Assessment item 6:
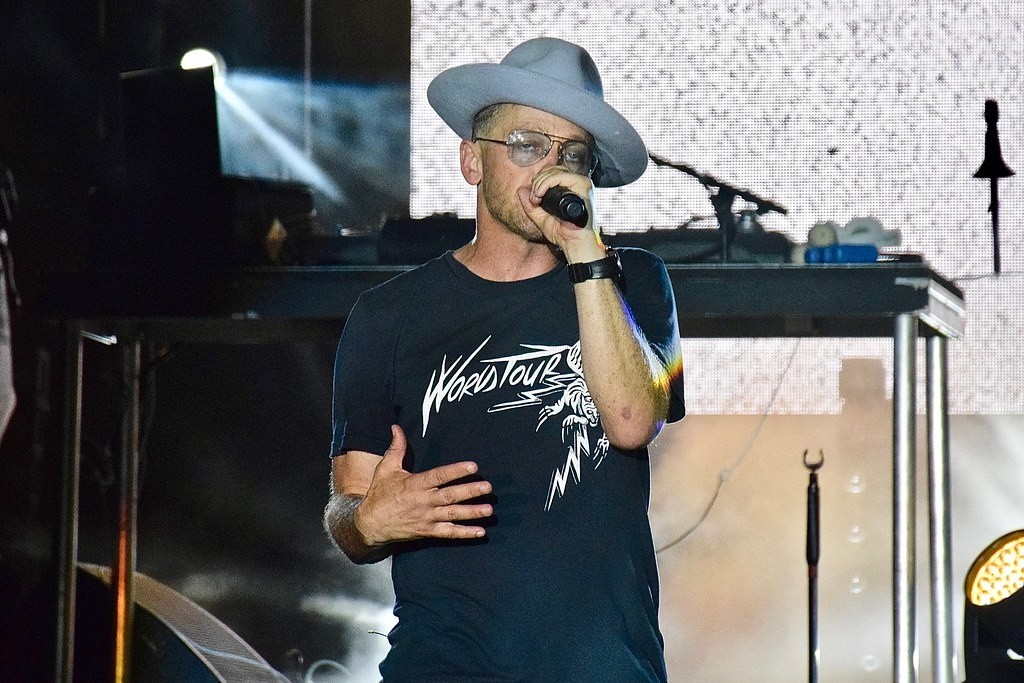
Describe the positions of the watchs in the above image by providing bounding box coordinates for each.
[568,245,623,284]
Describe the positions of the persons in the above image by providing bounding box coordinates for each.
[324,38,685,683]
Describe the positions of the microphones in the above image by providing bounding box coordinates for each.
[539,185,586,222]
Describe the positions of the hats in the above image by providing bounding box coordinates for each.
[426,36,648,188]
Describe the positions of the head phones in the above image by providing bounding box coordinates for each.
[806,214,901,246]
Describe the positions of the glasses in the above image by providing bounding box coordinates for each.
[473,130,599,178]
